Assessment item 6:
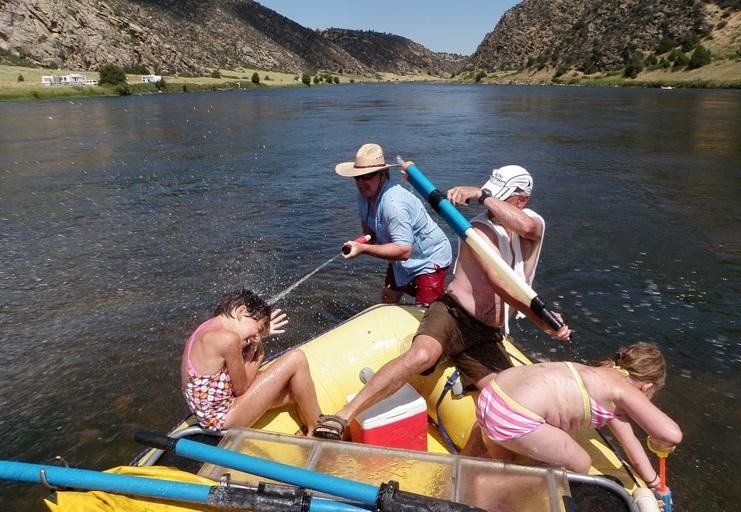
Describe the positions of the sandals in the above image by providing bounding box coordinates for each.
[313,411,348,442]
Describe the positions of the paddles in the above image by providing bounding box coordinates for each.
[132,429,490,512]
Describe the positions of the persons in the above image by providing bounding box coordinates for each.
[335,144,452,308]
[313,165,571,441]
[180,289,324,437]
[474,341,684,509]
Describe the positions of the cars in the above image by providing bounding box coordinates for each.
[82,79,97,85]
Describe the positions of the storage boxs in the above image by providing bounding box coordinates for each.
[347,382,428,464]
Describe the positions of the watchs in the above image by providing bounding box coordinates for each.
[479,187,491,204]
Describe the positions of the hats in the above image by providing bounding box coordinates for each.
[334,143,402,179]
[480,164,533,206]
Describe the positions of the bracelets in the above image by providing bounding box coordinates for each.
[645,474,658,484]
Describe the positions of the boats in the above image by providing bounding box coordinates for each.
[94,294,668,512]
[659,86,673,89]
